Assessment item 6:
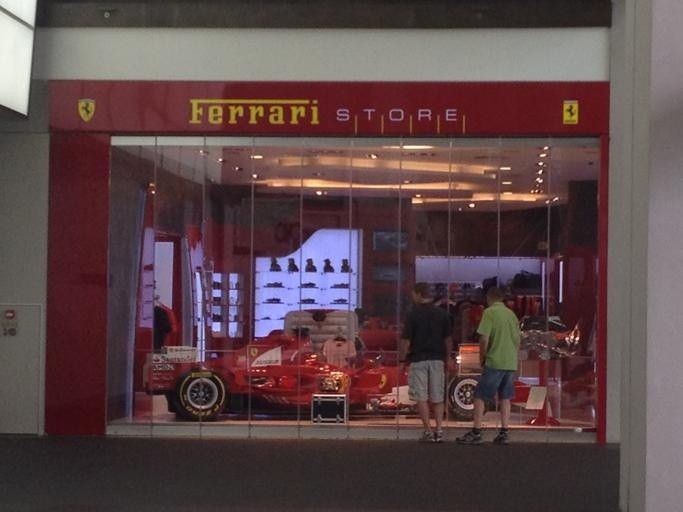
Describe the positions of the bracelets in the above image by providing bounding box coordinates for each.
[400,359,405,363]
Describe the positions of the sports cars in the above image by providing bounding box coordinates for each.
[146,330,540,424]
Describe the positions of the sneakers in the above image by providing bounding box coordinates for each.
[455,427,486,446]
[417,429,444,445]
[493,430,509,446]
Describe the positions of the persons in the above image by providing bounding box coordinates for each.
[431,285,455,309]
[454,286,522,446]
[397,280,451,443]
[446,302,476,374]
